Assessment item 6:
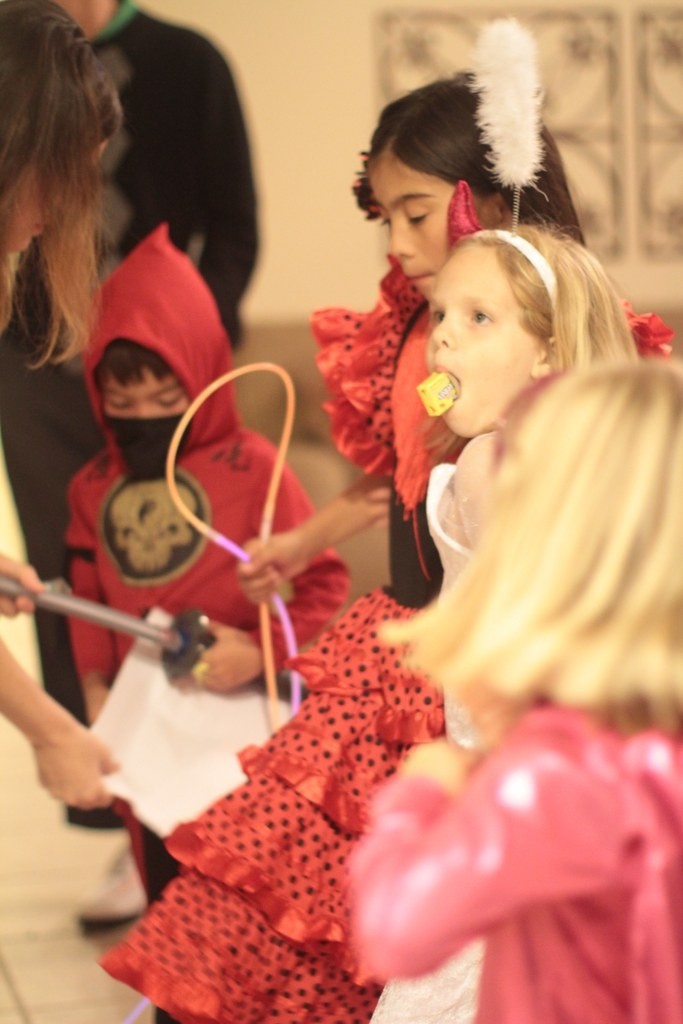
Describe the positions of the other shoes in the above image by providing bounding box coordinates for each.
[74,825,148,931]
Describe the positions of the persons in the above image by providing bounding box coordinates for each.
[95,69,675,1024]
[64,222,354,1024]
[347,365,683,1024]
[1,0,260,729]
[0,2,121,811]
[373,227,642,1024]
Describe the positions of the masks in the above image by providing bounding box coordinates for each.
[104,411,194,483]
